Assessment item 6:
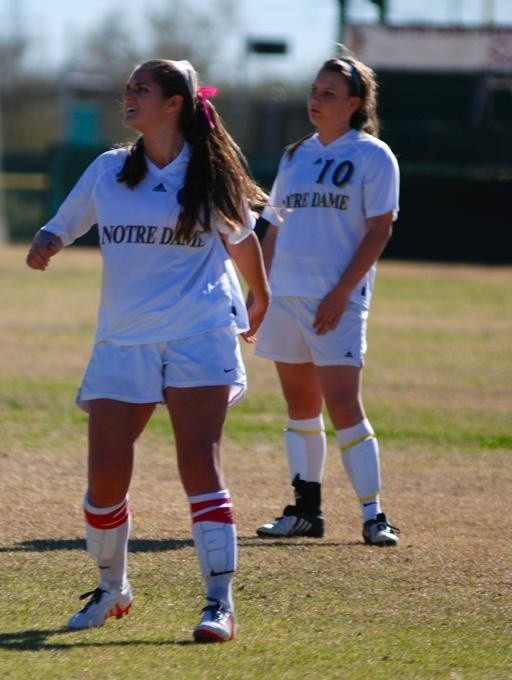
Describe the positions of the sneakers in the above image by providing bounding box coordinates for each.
[362,513,399,546]
[68,583,133,627]
[193,598,235,642]
[256,505,324,537]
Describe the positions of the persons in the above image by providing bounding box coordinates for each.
[26,59,271,642]
[240,56,400,545]
